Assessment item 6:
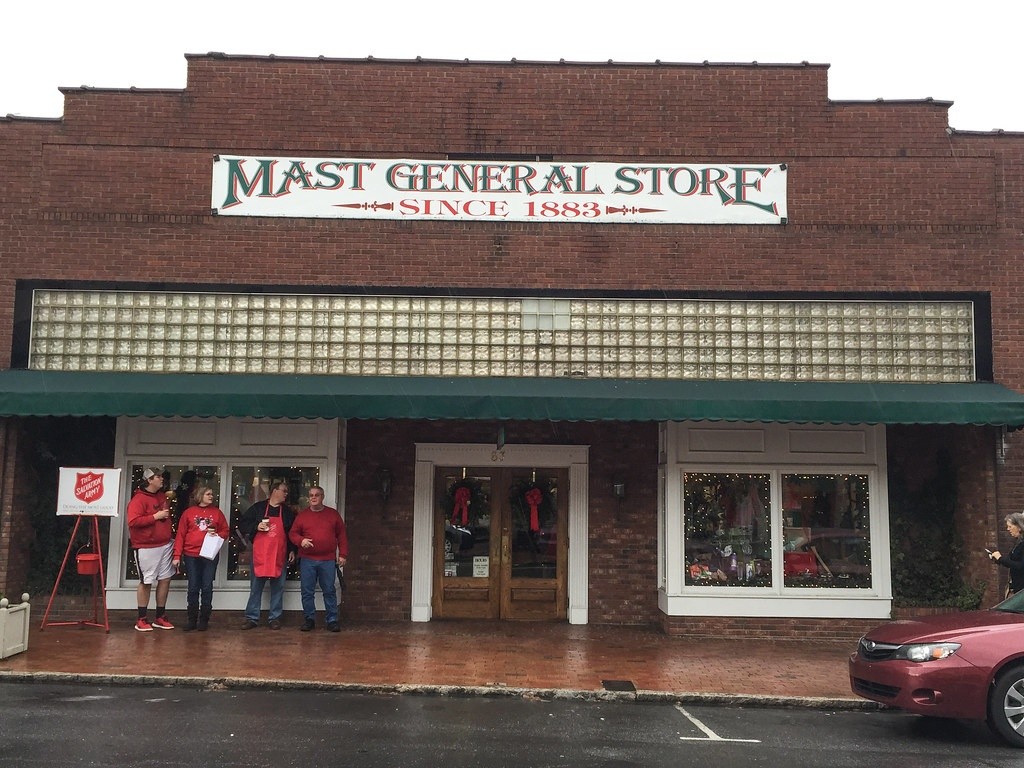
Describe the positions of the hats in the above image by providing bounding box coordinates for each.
[142,467,165,480]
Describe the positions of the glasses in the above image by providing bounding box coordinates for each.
[309,494,322,497]
[278,489,288,493]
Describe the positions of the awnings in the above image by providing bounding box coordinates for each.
[0,370,1024,427]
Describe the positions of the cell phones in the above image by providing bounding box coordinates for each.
[985,549,993,555]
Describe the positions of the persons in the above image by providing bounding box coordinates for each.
[172,484,230,631]
[987,512,1024,600]
[126,465,177,632]
[238,480,296,631]
[288,486,348,633]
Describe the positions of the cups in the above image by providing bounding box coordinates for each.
[163,508,169,519]
[208,527,215,534]
[262,519,269,531]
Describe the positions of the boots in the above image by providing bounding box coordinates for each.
[199,605,212,631]
[183,606,199,631]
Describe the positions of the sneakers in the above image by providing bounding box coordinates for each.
[328,622,341,632]
[303,619,315,631]
[241,620,257,630]
[271,622,280,630]
[152,615,174,629]
[135,616,153,631]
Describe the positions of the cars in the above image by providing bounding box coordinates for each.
[850,582,1024,751]
[756,530,870,587]
[533,518,557,564]
[445,519,473,555]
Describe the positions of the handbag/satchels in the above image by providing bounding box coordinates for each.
[1005,582,1015,600]
